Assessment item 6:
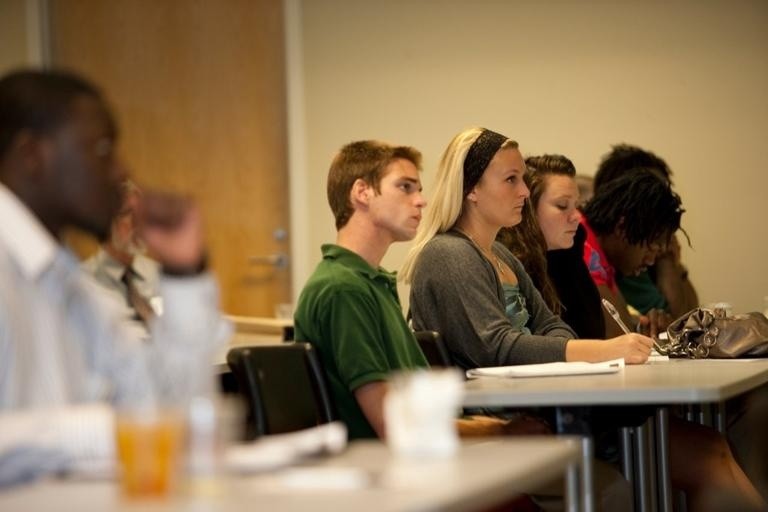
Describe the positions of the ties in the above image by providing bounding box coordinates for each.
[122,268,158,334]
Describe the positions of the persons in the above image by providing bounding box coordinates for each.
[0,62,228,489]
[294,140,630,512]
[397,127,767,510]
[519,142,767,492]
[82,180,160,327]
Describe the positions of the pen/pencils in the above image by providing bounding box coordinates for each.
[601,299,630,334]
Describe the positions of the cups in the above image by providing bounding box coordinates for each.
[116,365,190,501]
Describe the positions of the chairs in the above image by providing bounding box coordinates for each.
[226,342,337,436]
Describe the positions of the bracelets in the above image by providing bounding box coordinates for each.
[159,252,212,277]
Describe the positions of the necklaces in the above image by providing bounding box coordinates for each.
[457,224,505,276]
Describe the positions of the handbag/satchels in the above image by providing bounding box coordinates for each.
[664,304,768,360]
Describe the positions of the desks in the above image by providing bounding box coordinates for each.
[1,434,582,511]
[456,359,768,511]
